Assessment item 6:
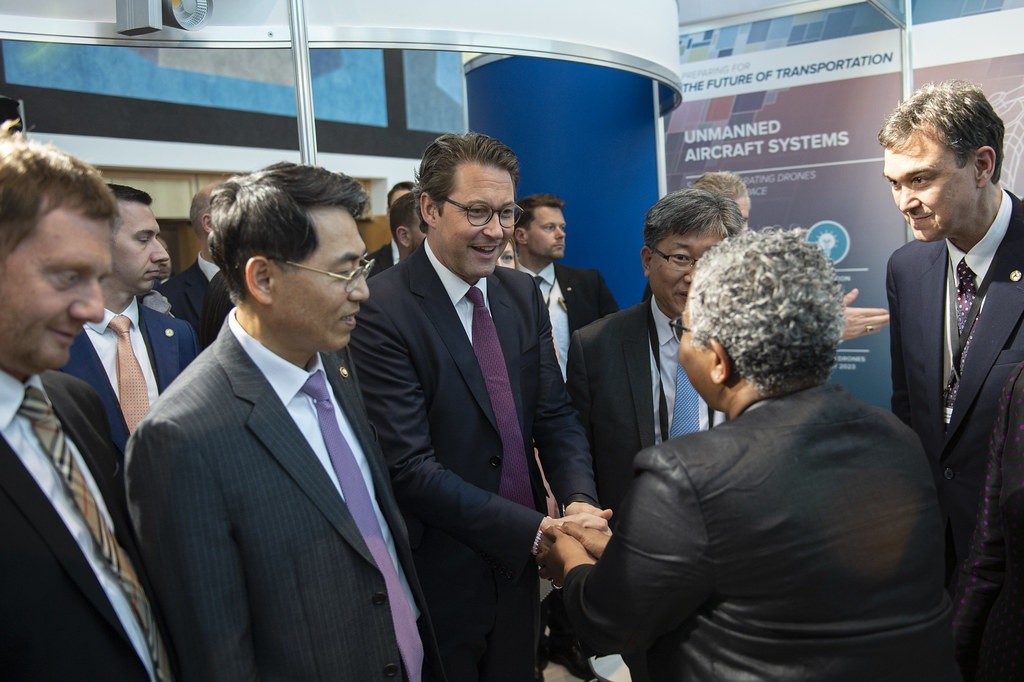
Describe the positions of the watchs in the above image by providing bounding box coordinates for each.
[562,494,602,517]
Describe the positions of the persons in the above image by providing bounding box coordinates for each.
[123,158,448,682]
[57,183,235,468]
[363,179,432,281]
[496,170,891,681]
[0,117,181,682]
[952,363,1024,682]
[876,78,1024,599]
[536,227,964,682]
[348,130,613,682]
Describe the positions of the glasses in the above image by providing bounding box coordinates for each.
[652,247,698,271]
[442,197,524,228]
[669,317,728,354]
[266,256,375,293]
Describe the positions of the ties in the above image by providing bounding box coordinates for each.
[671,363,700,440]
[535,277,545,302]
[944,257,980,435]
[299,370,424,682]
[108,315,150,436]
[464,287,536,511]
[16,386,174,682]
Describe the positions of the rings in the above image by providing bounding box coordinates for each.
[551,580,563,590]
[865,325,874,334]
